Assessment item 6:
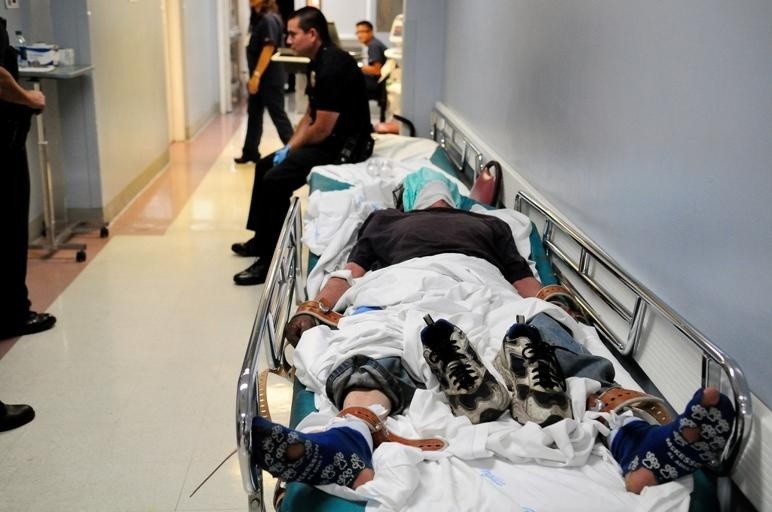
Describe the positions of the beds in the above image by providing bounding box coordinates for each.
[297,101,484,286]
[235,190,753,512]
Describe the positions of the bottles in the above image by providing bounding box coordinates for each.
[14,30,28,68]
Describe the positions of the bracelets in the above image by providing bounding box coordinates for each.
[253,69,261,79]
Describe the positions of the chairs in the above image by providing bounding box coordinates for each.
[468,160,502,207]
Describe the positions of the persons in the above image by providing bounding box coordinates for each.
[275,1,297,93]
[1,401,35,431]
[356,22,387,106]
[246,167,732,494]
[235,0,295,164]
[232,7,375,285]
[1,17,57,341]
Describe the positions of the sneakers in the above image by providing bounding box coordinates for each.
[418,316,510,423]
[231,234,271,257]
[490,314,572,431]
[1,398,35,433]
[235,254,270,286]
[0,308,57,341]
